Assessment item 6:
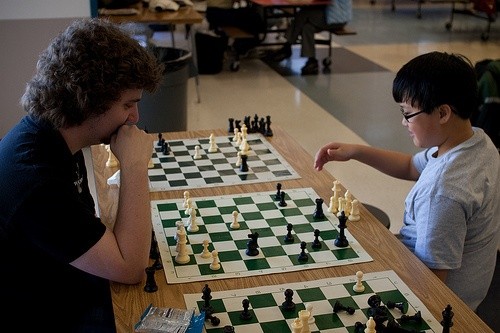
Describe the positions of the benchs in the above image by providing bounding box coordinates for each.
[221,8,357,72]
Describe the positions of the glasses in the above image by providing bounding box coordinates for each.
[400,108,434,122]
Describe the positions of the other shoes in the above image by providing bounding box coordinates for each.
[301,60,319,75]
[274,48,291,59]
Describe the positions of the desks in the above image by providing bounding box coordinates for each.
[91,128,494,333]
[100,8,204,104]
[251,0,333,17]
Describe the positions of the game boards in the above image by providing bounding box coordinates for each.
[149,184,373,284]
[182,269,451,333]
[147,124,303,192]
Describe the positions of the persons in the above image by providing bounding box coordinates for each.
[266,0,352,74]
[313,51,500,312]
[206,0,262,55]
[0,21,154,333]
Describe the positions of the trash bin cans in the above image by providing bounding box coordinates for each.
[129,47,191,133]
